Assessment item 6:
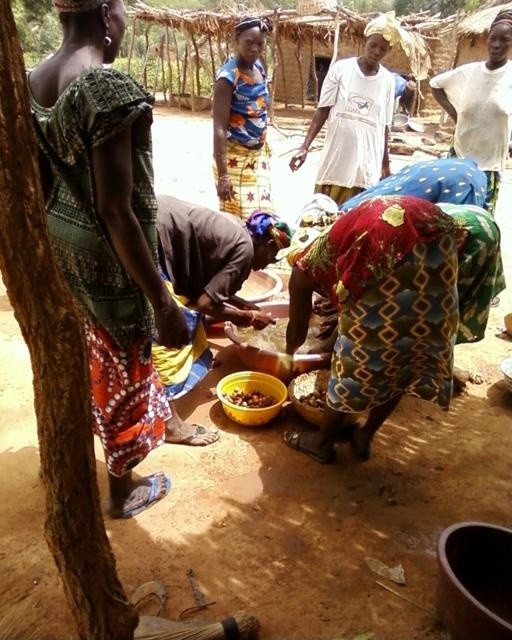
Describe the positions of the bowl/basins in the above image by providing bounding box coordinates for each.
[224,301,331,383]
[435,519,511,639]
[227,267,284,302]
[216,370,289,427]
[289,369,360,425]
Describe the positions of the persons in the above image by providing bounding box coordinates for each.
[144,193,293,447]
[294,156,489,317]
[295,202,507,345]
[289,10,402,204]
[429,9,511,222]
[212,16,275,222]
[25,0,192,519]
[275,194,462,464]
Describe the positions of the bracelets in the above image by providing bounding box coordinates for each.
[249,310,255,325]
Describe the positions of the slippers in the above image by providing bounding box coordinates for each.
[114,472,173,508]
[169,413,226,450]
[282,422,339,462]
